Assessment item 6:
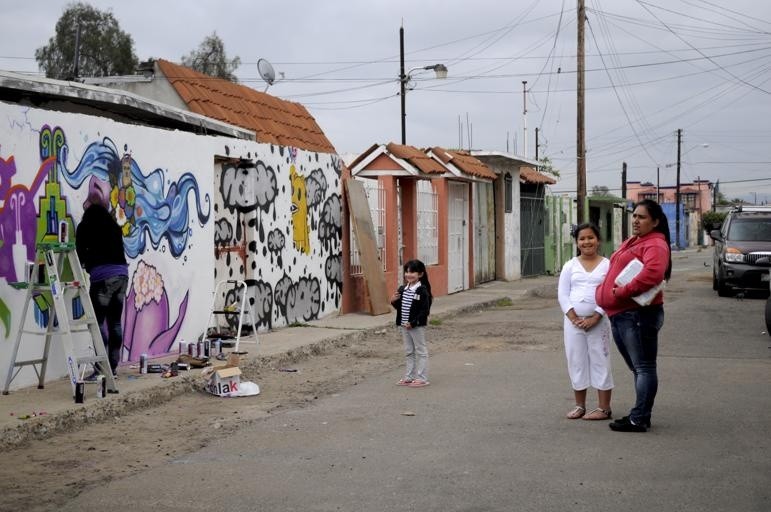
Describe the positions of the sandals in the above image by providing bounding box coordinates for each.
[567,406,612,420]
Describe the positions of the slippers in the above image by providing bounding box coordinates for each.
[396,378,430,387]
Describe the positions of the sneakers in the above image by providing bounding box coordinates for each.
[85,369,118,383]
[609,415,651,433]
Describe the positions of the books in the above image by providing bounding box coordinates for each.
[614,256,667,307]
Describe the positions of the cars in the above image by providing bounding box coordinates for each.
[711,206,771,293]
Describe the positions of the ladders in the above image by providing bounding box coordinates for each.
[202,279,260,354]
[3,243,120,404]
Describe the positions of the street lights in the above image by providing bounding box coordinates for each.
[399,63,449,145]
[676,142,710,249]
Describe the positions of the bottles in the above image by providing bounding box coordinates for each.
[175,364,190,370]
[139,352,147,375]
[178,338,223,361]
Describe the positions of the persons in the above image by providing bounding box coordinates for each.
[595,198,673,433]
[75,191,130,384]
[390,259,433,387]
[557,222,616,422]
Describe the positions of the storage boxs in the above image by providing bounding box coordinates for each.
[205,354,243,396]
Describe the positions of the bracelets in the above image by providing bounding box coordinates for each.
[572,319,578,324]
